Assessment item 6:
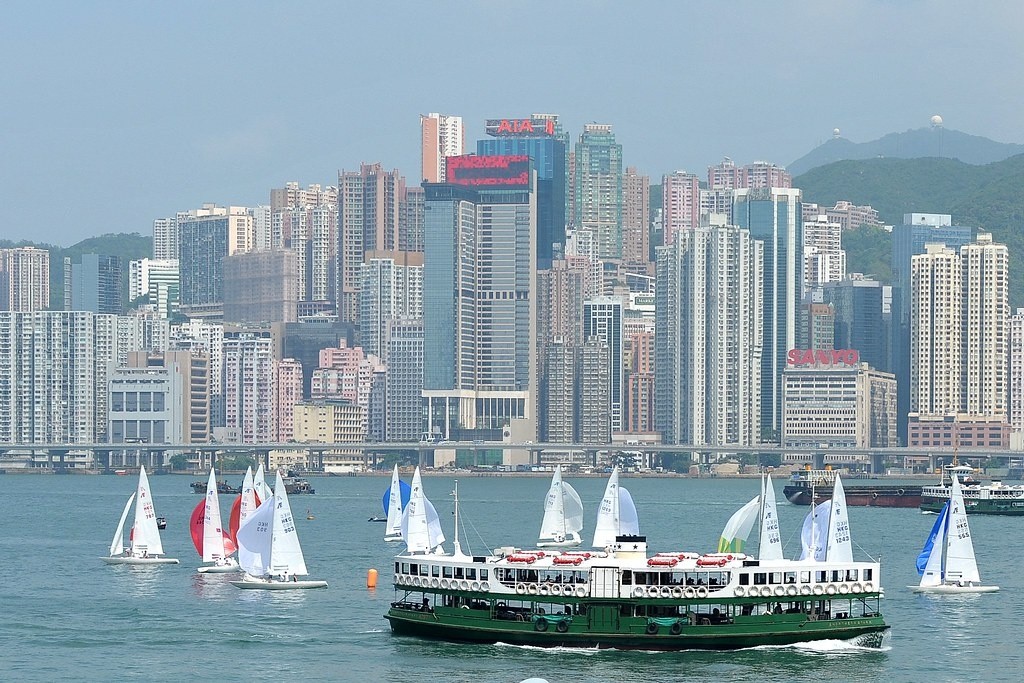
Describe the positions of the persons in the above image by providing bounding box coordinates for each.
[425,547,429,554]
[278,571,297,582]
[125,548,149,558]
[604,544,616,557]
[707,602,800,625]
[421,574,588,613]
[554,535,563,542]
[652,577,796,585]
[215,558,226,566]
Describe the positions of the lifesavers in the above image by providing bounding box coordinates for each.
[748,586,759,597]
[800,584,812,596]
[760,585,772,597]
[774,585,785,596]
[851,582,862,593]
[394,574,491,592]
[825,583,838,595]
[734,585,746,596]
[863,582,875,593]
[536,618,683,635]
[634,585,708,598]
[812,584,824,595]
[786,585,798,596]
[838,583,850,594]
[516,582,586,597]
